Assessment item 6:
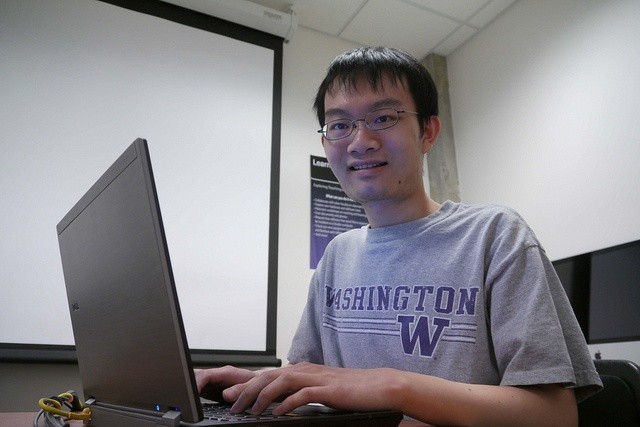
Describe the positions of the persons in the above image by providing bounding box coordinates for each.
[193,46,603,426]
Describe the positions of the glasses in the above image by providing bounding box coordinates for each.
[318,107,420,141]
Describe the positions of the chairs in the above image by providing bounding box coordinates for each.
[578,360,636,425]
